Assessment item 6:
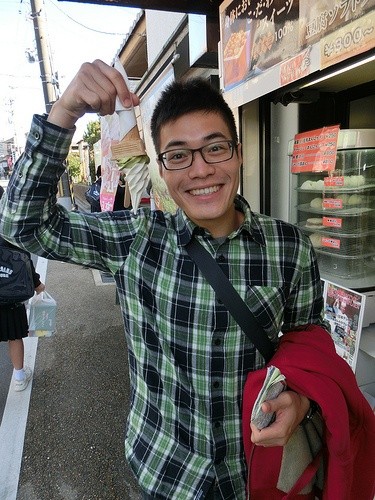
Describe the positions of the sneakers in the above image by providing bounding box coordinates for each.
[13,366,32,391]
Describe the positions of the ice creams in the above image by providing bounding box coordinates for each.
[110,104,151,215]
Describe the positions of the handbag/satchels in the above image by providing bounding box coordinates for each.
[27,291,56,337]
[0,247,33,303]
[303,410,328,491]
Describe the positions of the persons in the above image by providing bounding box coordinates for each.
[0,60,330,500]
[0,186,45,391]
[334,299,348,341]
[86,165,132,277]
[0,169,12,180]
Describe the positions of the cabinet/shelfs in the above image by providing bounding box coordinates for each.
[287,129,375,289]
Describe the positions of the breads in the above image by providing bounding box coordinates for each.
[310,194,361,207]
[301,175,364,190]
[305,218,327,229]
[309,233,330,247]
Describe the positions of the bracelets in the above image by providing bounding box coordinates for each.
[300,400,315,425]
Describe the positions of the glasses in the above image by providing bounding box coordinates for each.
[158,140,238,171]
